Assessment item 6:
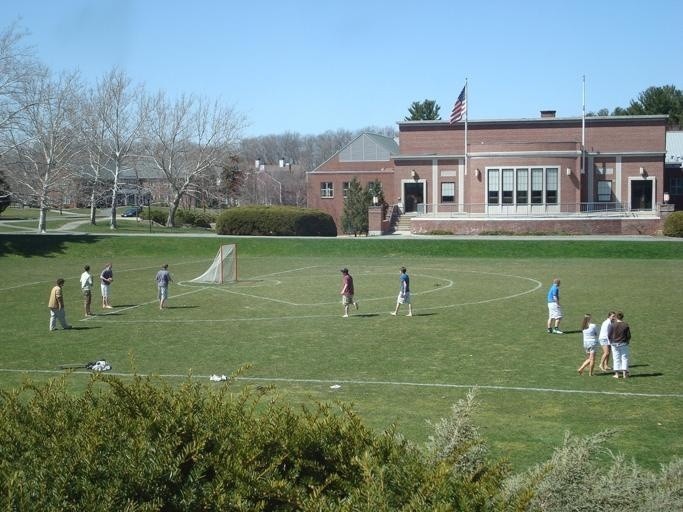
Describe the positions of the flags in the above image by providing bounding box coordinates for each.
[449,82,466,129]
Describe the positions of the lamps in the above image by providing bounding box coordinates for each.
[410,169,416,179]
[639,167,645,175]
[566,167,572,176]
[372,194,379,207]
[474,167,480,177]
[663,192,671,204]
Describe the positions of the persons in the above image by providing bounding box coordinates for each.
[606,311,631,379]
[410,194,417,212]
[78,265,94,316]
[575,313,598,376]
[389,266,413,318]
[595,311,616,372]
[339,267,358,318]
[99,262,114,309]
[154,264,171,309]
[47,277,73,331]
[546,278,565,333]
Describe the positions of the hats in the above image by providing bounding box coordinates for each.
[340,269,348,273]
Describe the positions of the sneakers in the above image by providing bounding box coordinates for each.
[547,329,551,333]
[342,315,348,318]
[354,303,359,310]
[406,313,412,316]
[391,312,396,316]
[552,329,563,334]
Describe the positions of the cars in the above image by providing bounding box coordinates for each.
[121,207,140,217]
